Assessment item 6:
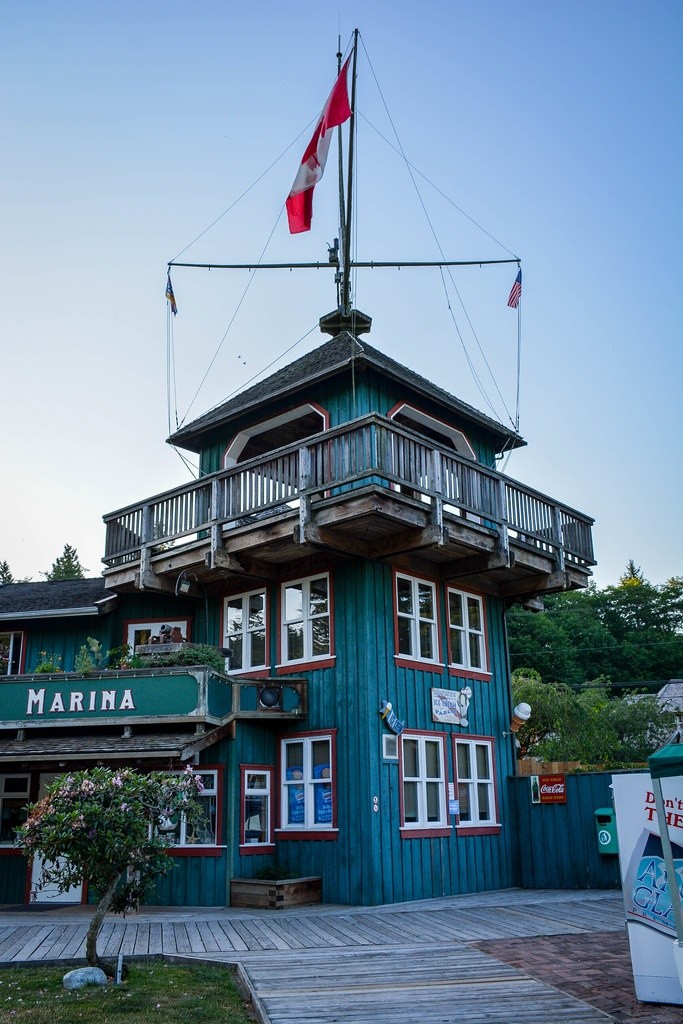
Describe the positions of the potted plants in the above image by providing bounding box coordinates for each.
[120,653,133,671]
[230,866,323,911]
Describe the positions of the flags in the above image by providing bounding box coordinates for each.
[286,48,350,236]
[508,270,521,308]
[165,276,177,316]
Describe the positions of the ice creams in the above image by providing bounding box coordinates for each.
[510,703,531,732]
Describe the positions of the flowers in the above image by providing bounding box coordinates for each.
[0,640,16,675]
[31,651,63,673]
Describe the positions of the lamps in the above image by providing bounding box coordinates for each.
[121,727,133,739]
[14,729,26,742]
[180,574,191,594]
